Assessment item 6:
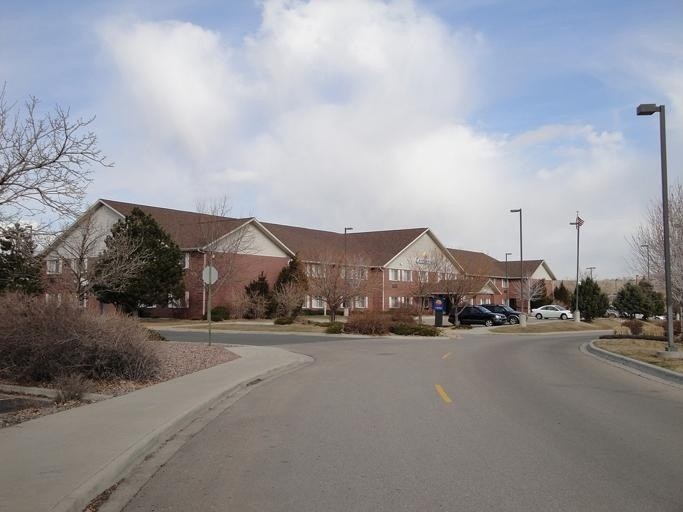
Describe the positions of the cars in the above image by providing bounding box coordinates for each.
[531,304,574,320]
[604,305,668,321]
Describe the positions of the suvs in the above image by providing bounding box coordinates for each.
[448,306,506,327]
[479,304,528,325]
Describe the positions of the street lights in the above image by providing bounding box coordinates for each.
[345,227,354,316]
[505,252,511,304]
[586,266,596,280]
[640,244,650,283]
[636,102,679,353]
[509,208,524,314]
